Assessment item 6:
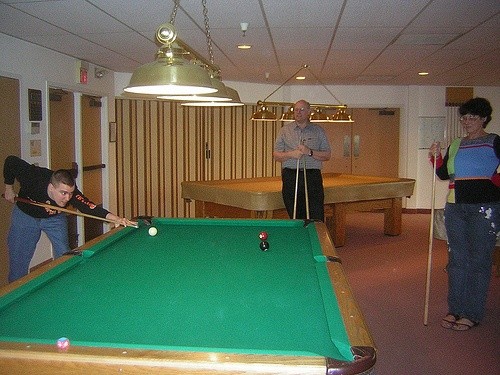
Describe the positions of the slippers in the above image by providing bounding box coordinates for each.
[453,317,480,331]
[441,313,460,330]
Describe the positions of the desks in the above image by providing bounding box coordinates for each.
[0,213,379,375]
[180,172,416,246]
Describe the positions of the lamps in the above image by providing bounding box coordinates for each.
[247,63,357,125]
[122,0,245,108]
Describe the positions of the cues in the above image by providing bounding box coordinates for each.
[1,194,139,228]
[302,132,310,219]
[423,140,437,325]
[292,137,301,219]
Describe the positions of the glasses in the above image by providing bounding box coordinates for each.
[294,107,309,111]
[458,114,481,122]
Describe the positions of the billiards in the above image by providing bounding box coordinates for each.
[259,241,270,251]
[258,231,268,240]
[148,226,158,236]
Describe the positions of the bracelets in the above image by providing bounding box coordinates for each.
[309,149,313,156]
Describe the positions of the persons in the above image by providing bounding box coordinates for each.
[428,97,500,332]
[273,99,331,223]
[4,154,138,283]
